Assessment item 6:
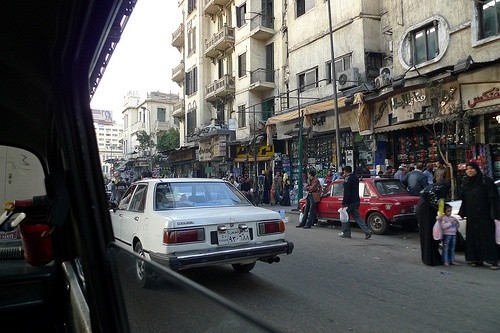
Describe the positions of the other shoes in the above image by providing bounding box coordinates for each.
[471,262,483,267]
[451,261,462,266]
[443,262,450,267]
[296,223,305,228]
[365,231,372,239]
[338,232,351,238]
[303,224,311,228]
[490,265,500,270]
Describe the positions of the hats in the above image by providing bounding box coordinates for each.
[399,166,403,168]
[401,164,407,167]
[434,157,440,162]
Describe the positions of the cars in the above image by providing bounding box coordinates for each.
[299,178,420,235]
[109,178,294,289]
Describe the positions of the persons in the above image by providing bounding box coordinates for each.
[416,179,450,267]
[222,167,292,207]
[440,203,462,267]
[324,159,450,196]
[295,168,322,229]
[458,162,500,270]
[338,166,372,240]
[110,176,131,202]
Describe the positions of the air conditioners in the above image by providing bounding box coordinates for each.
[375,65,394,89]
[336,68,359,90]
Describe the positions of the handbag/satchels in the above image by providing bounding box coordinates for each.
[494,219,500,245]
[433,219,443,240]
[338,207,349,223]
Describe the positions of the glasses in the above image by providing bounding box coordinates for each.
[432,162,439,164]
